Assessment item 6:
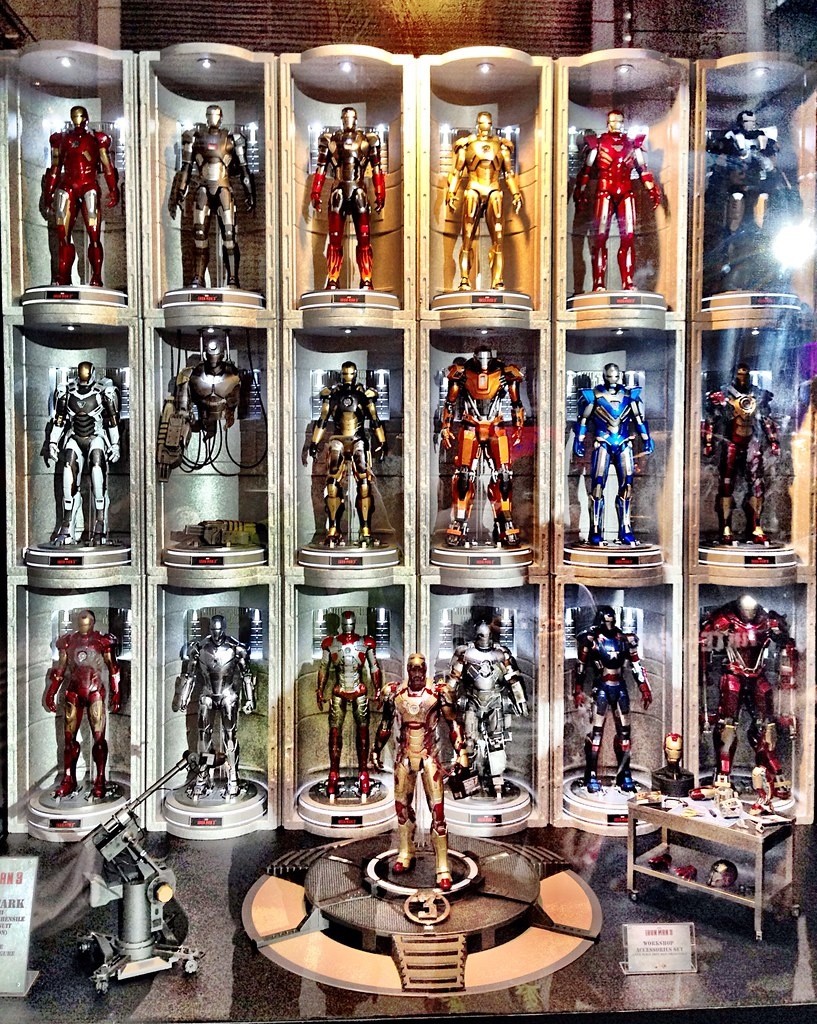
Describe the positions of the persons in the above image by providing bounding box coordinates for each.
[446,111,523,291]
[703,363,782,545]
[441,346,525,545]
[700,595,795,816]
[45,610,120,798]
[574,363,655,544]
[573,110,661,292]
[705,110,791,290]
[372,653,468,892]
[43,106,117,287]
[176,105,253,289]
[571,606,652,793]
[651,733,694,797]
[311,107,386,291]
[309,361,386,547]
[317,611,382,797]
[173,616,256,795]
[158,340,255,545]
[48,362,122,544]
[446,623,529,799]
